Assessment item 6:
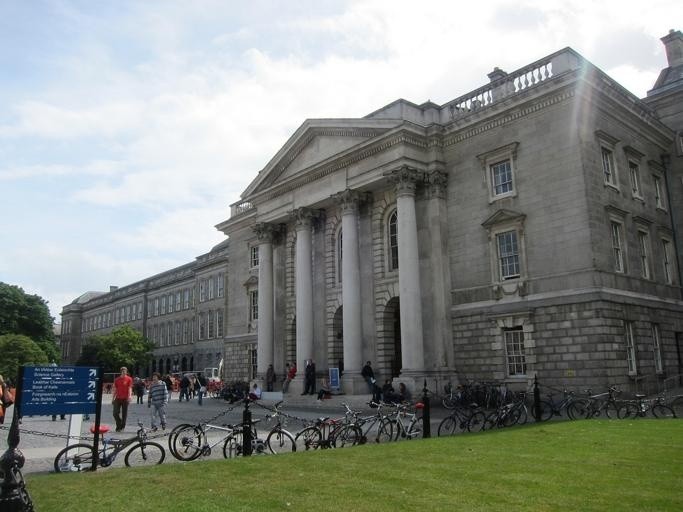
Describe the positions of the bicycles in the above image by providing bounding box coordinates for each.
[438,379,528,436]
[53,417,165,472]
[531,386,676,422]
[169,400,424,462]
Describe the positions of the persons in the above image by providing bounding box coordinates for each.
[285,362,290,374]
[300,359,315,393]
[103,364,224,403]
[110,366,133,432]
[281,362,297,393]
[382,377,393,403]
[316,375,334,399]
[266,364,275,391]
[51,413,66,421]
[361,359,377,392]
[394,381,411,403]
[246,382,262,405]
[0,376,6,424]
[3,376,16,392]
[147,372,168,431]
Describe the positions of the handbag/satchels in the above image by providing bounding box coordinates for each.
[199,386,207,393]
[142,387,147,395]
